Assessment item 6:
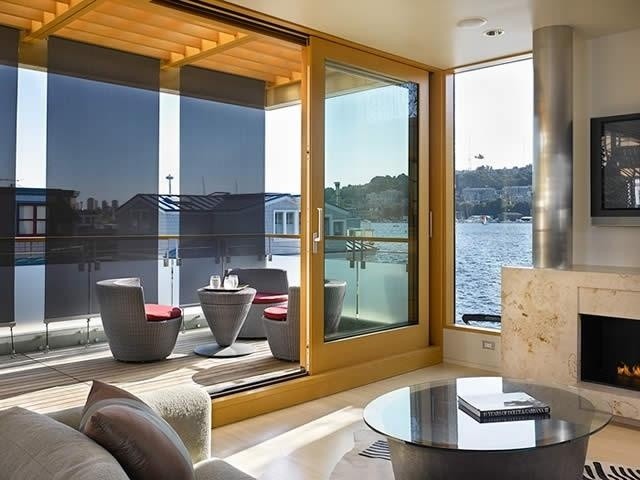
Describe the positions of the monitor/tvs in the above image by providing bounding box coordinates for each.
[590,112,640,227]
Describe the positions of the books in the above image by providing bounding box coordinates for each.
[457,403,550,423]
[457,391,550,417]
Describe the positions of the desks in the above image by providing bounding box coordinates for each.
[193,286,257,357]
[362,376,614,480]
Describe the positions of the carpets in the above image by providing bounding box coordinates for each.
[326,428,640,479]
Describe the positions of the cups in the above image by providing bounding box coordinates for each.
[210,274,239,290]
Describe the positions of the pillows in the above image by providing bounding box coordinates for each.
[78,380,194,480]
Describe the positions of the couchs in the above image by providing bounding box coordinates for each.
[0,380,254,479]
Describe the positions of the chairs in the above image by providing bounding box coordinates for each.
[261,278,347,361]
[226,268,289,338]
[95,277,183,363]
